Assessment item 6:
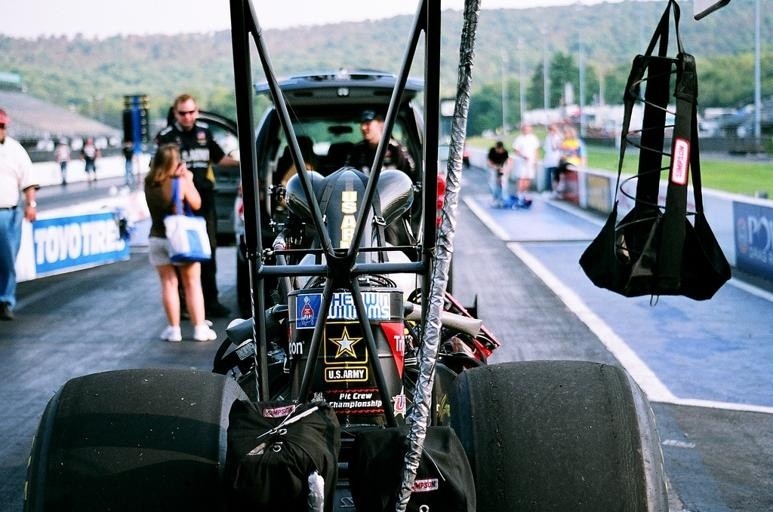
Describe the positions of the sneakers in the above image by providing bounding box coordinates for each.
[0,300,16,322]
[192,323,217,341]
[160,325,181,342]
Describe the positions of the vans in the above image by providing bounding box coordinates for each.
[167,66,456,318]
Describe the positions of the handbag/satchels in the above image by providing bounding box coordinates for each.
[163,214,212,262]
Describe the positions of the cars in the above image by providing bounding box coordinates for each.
[564,100,773,141]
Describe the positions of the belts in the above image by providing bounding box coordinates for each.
[0,205,18,210]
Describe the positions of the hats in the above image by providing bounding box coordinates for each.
[0,108,11,125]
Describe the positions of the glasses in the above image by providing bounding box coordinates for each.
[178,110,195,115]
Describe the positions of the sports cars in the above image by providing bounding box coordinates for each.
[23,0,671,511]
